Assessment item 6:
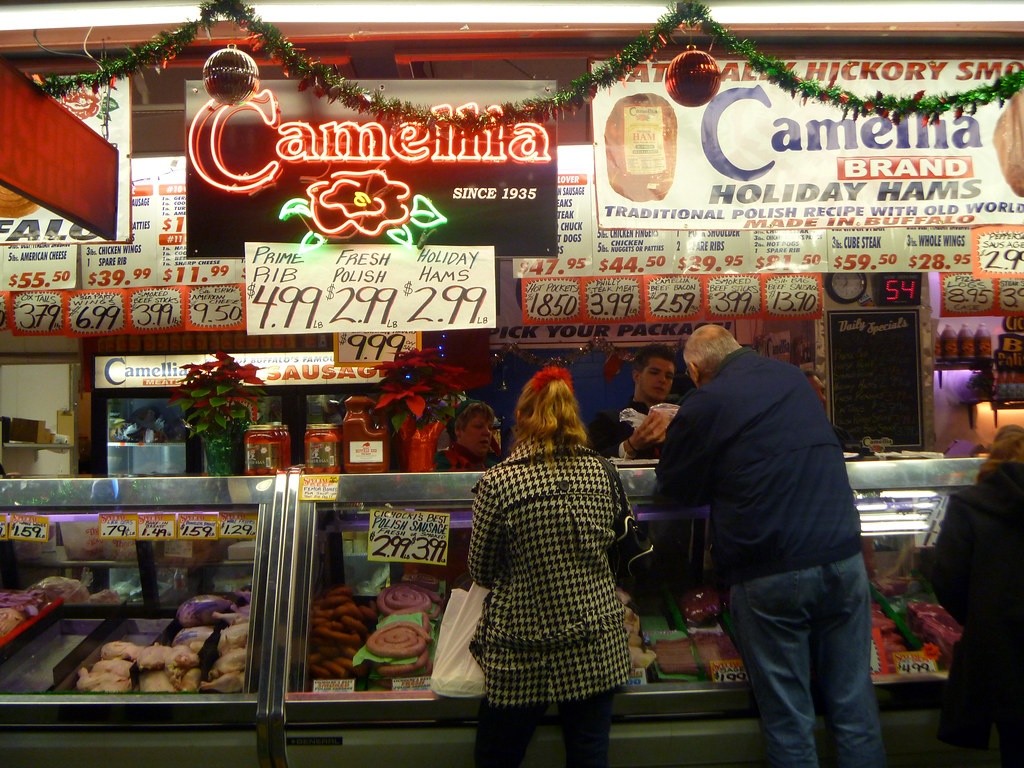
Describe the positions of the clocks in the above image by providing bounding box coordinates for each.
[824,273,867,304]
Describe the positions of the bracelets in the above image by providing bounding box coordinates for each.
[628,437,639,452]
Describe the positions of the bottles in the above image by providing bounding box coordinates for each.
[935,323,992,361]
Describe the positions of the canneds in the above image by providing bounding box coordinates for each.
[243,422,341,476]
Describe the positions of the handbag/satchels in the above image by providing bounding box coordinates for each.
[597,456,654,586]
[430,582,491,698]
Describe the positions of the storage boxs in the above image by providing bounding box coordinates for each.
[8,417,47,444]
[106,442,188,476]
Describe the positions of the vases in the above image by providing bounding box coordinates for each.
[201,416,242,475]
[390,411,450,474]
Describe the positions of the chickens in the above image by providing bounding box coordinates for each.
[77,611,250,694]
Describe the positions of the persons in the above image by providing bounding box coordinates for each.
[468,367,635,768]
[804,370,827,407]
[925,424,1024,768]
[587,346,674,458]
[432,400,498,471]
[658,321,884,768]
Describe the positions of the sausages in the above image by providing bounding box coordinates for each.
[304,572,446,679]
[870,601,906,673]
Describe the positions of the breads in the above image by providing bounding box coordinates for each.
[991,88,1024,198]
[604,92,678,202]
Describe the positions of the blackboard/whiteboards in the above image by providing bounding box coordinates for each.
[825,307,924,448]
[243,240,498,335]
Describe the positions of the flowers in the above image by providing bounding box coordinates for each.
[372,347,495,434]
[166,346,268,436]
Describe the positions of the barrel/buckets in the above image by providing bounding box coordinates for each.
[343,395,390,474]
